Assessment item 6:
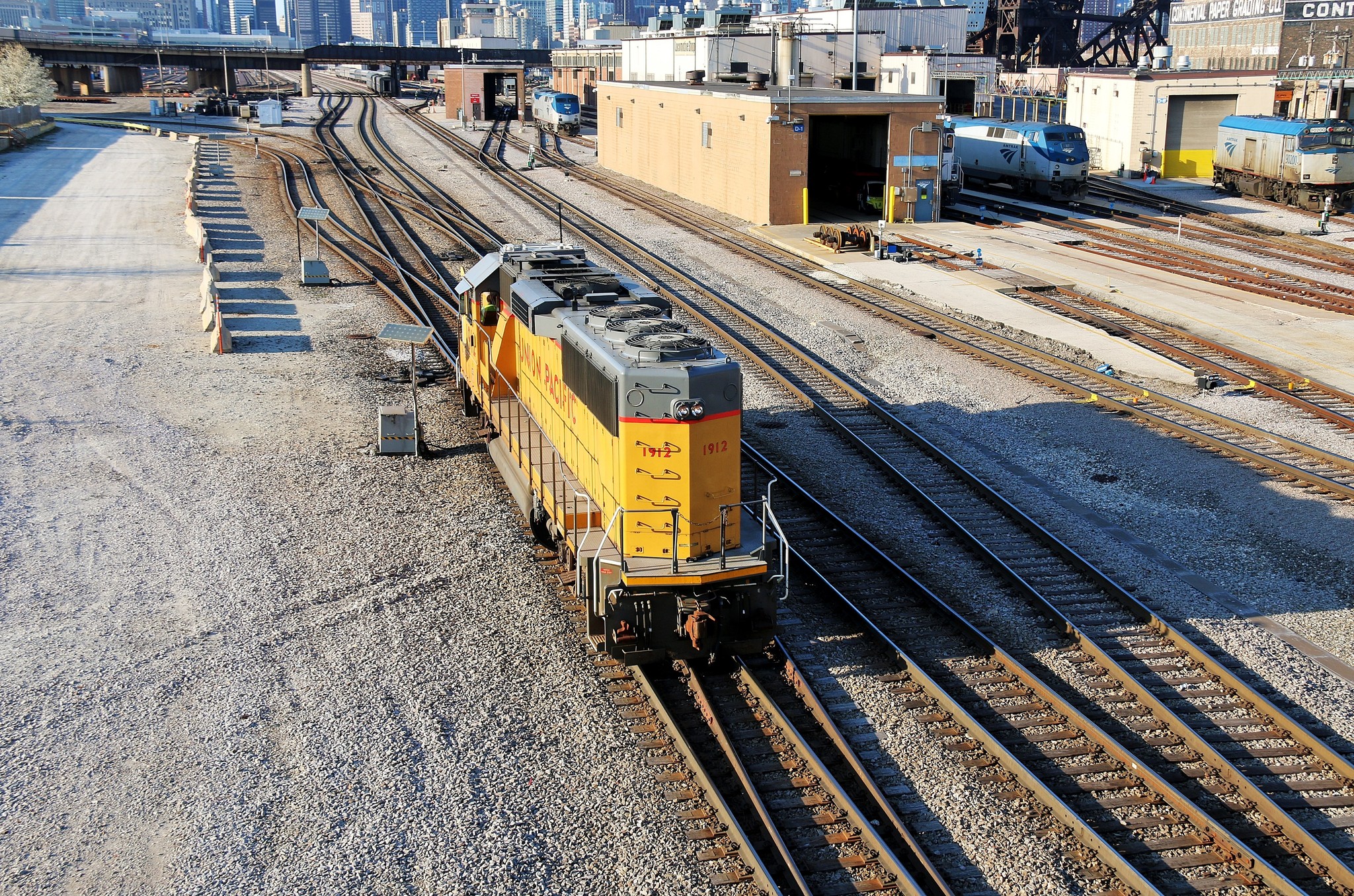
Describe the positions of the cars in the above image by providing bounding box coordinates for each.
[857,180,885,213]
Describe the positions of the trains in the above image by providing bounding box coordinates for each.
[454,237,785,666]
[531,87,581,136]
[334,67,392,98]
[1212,112,1354,214]
[943,113,1089,202]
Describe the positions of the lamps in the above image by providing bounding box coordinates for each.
[921,163,932,172]
[793,116,804,125]
[1128,69,1138,80]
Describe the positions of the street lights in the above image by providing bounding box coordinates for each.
[421,20,426,40]
[219,48,230,98]
[154,2,164,45]
[458,48,468,132]
[154,47,165,107]
[323,13,330,45]
[292,18,298,49]
[263,20,268,48]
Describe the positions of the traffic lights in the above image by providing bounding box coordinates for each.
[1324,213,1329,223]
